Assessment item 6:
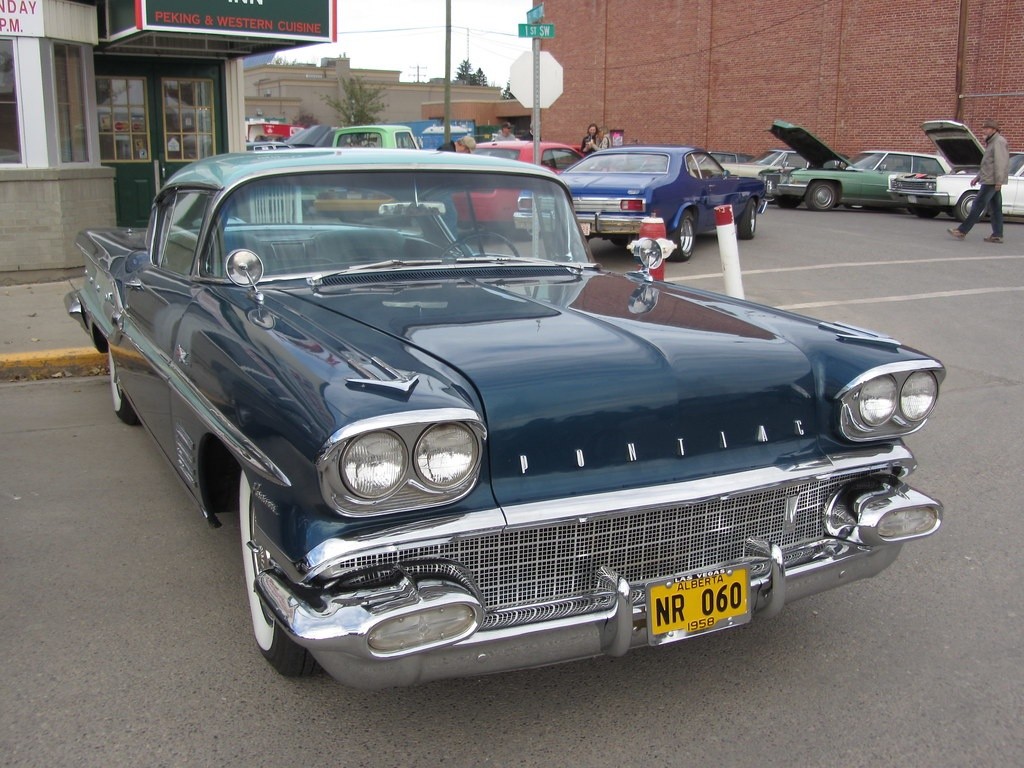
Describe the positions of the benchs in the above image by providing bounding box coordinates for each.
[162,228,407,278]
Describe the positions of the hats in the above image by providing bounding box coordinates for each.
[981,120,999,131]
[462,136,477,154]
[502,122,514,128]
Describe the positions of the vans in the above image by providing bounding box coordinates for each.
[246,143,295,150]
[332,126,421,152]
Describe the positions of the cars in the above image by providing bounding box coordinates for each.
[451,139,587,228]
[514,151,770,264]
[314,185,401,222]
[768,120,954,211]
[62,143,946,691]
[886,120,1024,221]
[718,148,810,203]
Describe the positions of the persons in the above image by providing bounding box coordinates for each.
[425,136,476,190]
[947,119,1009,243]
[520,121,541,142]
[495,122,516,141]
[581,124,611,152]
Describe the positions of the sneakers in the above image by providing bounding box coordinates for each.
[984,234,1004,243]
[948,227,966,240]
